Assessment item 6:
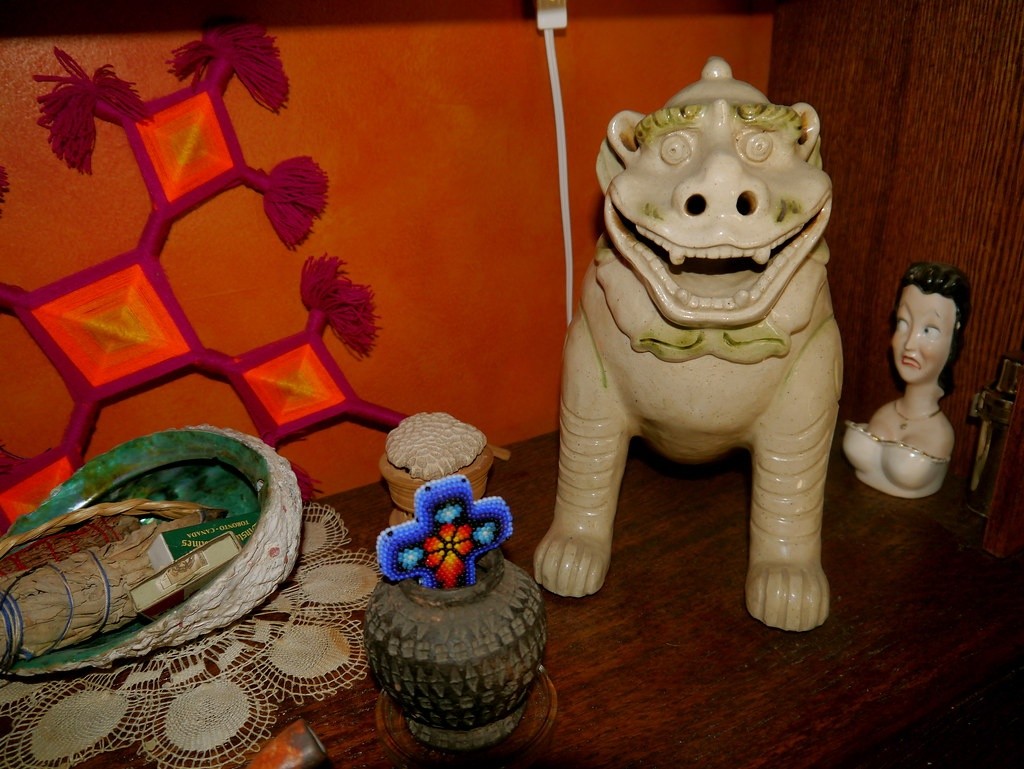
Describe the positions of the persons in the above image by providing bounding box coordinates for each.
[841,260,972,498]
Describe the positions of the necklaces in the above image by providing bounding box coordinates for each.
[895,397,943,430]
